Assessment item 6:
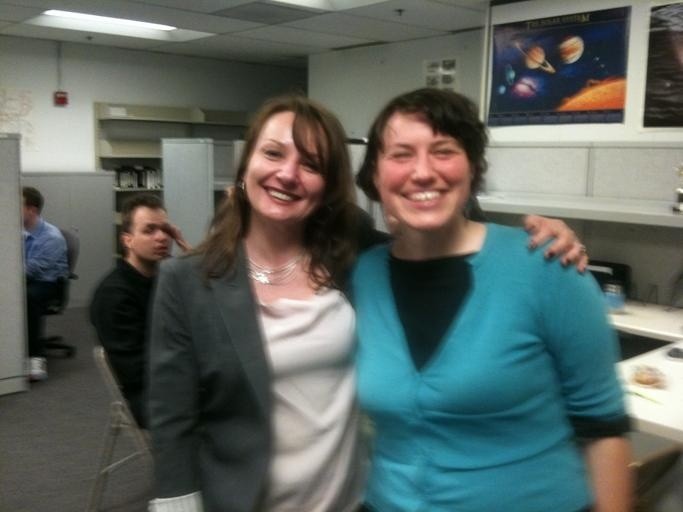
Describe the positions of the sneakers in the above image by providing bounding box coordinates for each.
[27,357,48,380]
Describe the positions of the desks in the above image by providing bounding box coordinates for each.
[611,297,683,445]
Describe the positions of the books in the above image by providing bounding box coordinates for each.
[117,165,163,189]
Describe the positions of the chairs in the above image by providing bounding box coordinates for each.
[33,227,159,480]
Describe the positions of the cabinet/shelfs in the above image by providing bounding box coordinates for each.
[96,104,262,228]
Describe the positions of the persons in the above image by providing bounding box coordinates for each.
[22,186,71,381]
[90,192,195,426]
